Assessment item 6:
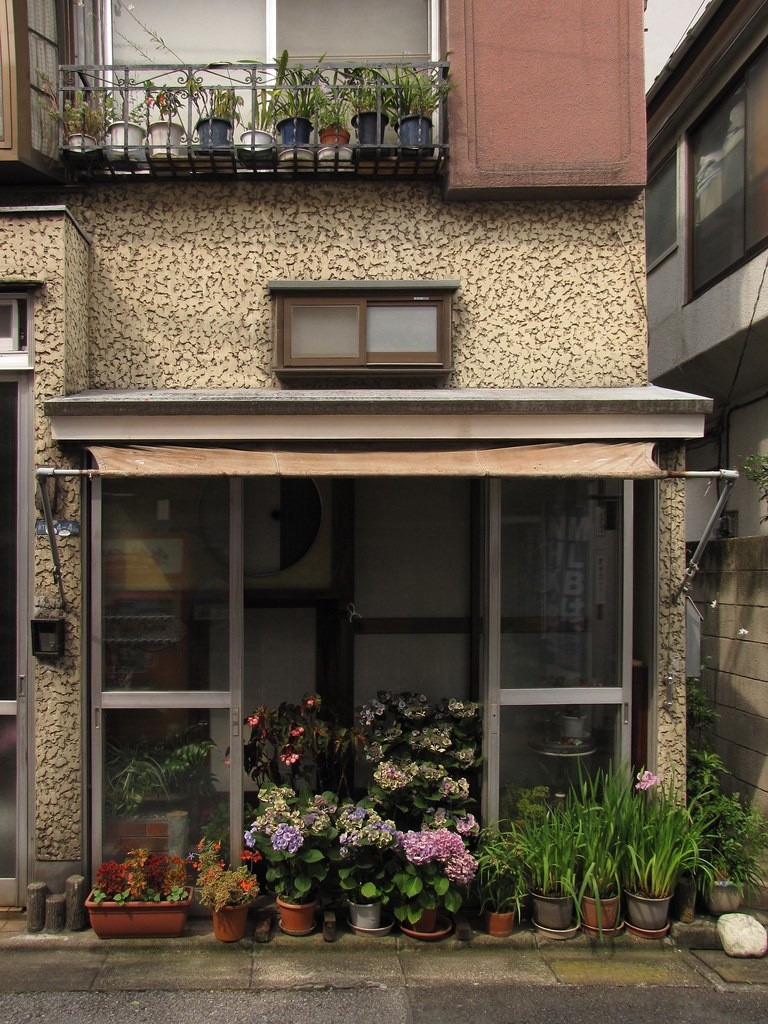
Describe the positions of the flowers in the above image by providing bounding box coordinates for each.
[623,772,720,899]
[88,691,483,927]
[146,91,183,122]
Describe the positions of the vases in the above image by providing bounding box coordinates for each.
[146,122,186,159]
[209,899,254,942]
[624,888,674,940]
[275,895,318,938]
[84,887,196,938]
[346,898,394,937]
[398,897,455,942]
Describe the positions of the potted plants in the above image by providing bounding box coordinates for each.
[34,67,154,162]
[103,725,220,815]
[188,50,461,167]
[682,790,768,912]
[561,702,589,738]
[464,760,644,944]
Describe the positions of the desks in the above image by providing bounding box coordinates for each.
[530,737,597,785]
